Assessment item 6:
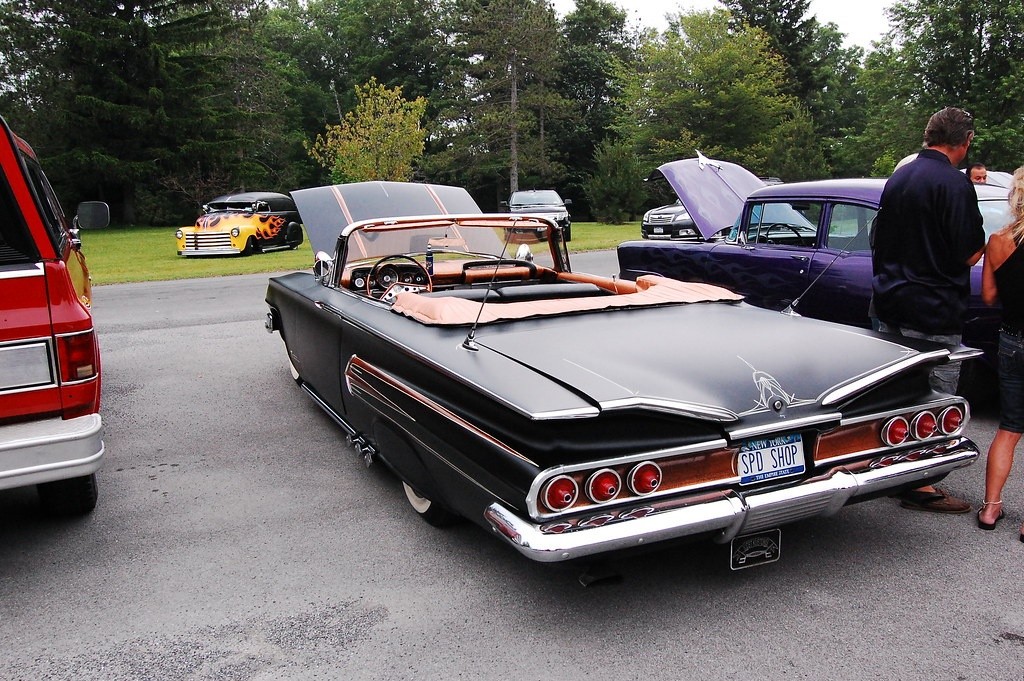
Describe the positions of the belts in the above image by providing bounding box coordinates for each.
[999,321,1024,338]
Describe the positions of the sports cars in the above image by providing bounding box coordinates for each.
[264,181,985,571]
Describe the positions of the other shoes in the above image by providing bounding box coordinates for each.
[900,488,972,513]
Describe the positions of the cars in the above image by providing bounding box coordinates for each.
[499,149,1024,412]
[0,113,109,513]
[176,192,303,259]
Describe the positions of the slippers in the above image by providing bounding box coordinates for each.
[977,506,1005,530]
[1020,534,1024,544]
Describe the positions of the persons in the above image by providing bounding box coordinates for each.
[976,166,1024,542]
[869,106,987,514]
[965,160,990,184]
